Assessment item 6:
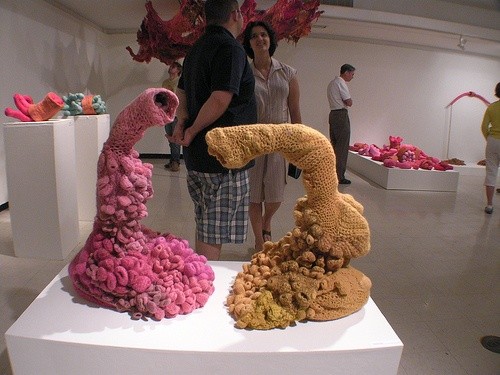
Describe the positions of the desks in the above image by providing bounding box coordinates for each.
[5,249,404,375]
[347,150,500,191]
[2,118,82,260]
[58,114,110,221]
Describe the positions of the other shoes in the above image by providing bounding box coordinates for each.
[338,179,351,184]
[485,207,493,214]
[164,163,171,168]
[171,161,179,171]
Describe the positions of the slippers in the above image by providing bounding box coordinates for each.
[263,230,272,242]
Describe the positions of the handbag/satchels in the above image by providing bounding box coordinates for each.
[288,162,302,179]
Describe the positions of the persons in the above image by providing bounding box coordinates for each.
[243,20,304,258]
[481,82,500,215]
[163,0,255,262]
[326,64,355,185]
[163,62,182,172]
